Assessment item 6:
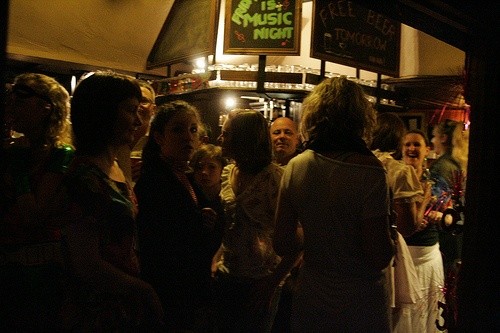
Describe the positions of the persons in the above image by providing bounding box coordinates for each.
[0,71,469,333]
